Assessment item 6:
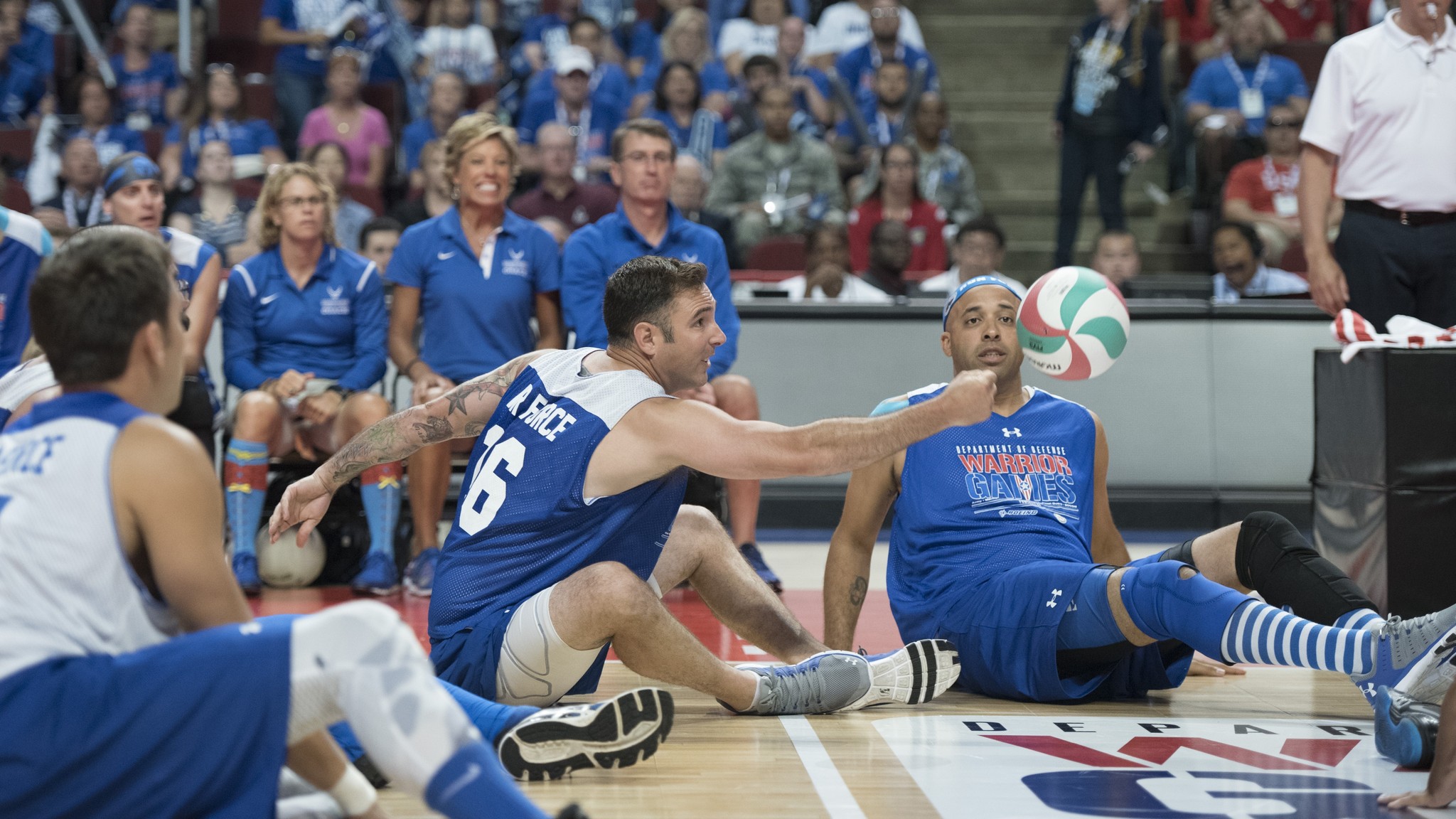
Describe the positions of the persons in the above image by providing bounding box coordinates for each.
[822,274,1456,768]
[1,1,1456,819]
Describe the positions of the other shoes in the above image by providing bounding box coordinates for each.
[738,541,783,594]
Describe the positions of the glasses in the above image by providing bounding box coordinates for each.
[1267,117,1301,128]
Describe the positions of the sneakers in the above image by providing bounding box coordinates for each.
[834,639,962,711]
[229,549,262,595]
[1373,686,1443,767]
[350,552,400,595]
[402,548,441,596]
[715,650,872,718]
[499,688,675,783]
[1349,603,1456,711]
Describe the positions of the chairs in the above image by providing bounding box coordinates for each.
[204,317,577,584]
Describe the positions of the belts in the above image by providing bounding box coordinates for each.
[1348,200,1456,227]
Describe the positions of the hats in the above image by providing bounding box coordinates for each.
[552,43,594,77]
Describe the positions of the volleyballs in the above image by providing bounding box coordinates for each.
[1014,265,1132,381]
[255,518,327,589]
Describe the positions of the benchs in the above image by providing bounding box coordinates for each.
[0,0,815,290]
[1160,42,1347,285]
